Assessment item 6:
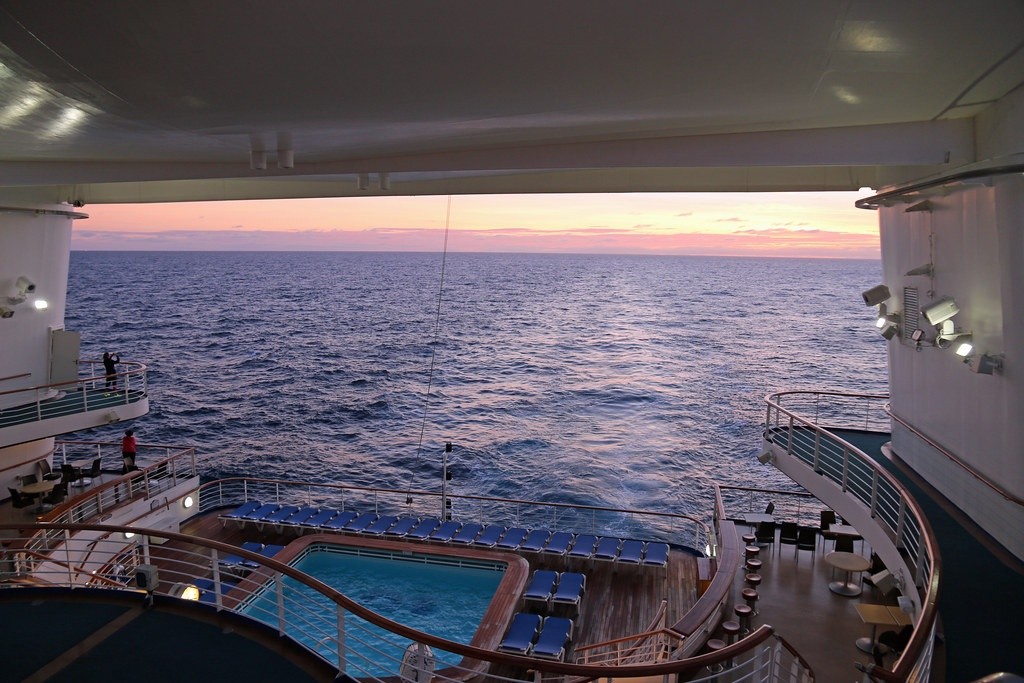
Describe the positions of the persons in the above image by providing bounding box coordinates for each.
[103,353,122,398]
[122,430,137,484]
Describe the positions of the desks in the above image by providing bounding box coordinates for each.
[829,523,866,553]
[695,557,719,601]
[886,605,914,627]
[852,603,898,656]
[824,551,872,597]
[742,514,775,534]
[19,481,55,516]
[69,459,92,488]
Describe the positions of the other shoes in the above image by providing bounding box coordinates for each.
[113,387,122,391]
[105,388,112,392]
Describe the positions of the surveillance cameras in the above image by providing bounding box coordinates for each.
[862,285,891,307]
[921,296,960,326]
[15,276,36,294]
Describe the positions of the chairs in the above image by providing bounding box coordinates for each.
[566,534,599,564]
[819,510,838,552]
[516,529,552,559]
[122,456,134,482]
[60,475,71,502]
[43,481,67,510]
[765,501,775,515]
[300,509,341,534]
[360,515,399,540]
[539,532,576,565]
[589,537,623,568]
[778,520,799,559]
[257,505,299,533]
[529,616,575,663]
[82,456,105,488]
[21,472,47,506]
[341,513,379,537]
[320,512,359,536]
[199,581,238,605]
[521,569,560,610]
[279,507,319,533]
[428,521,464,545]
[238,545,288,577]
[407,518,441,544]
[642,542,671,577]
[754,521,776,552]
[549,572,587,614]
[498,611,544,655]
[39,458,62,483]
[495,527,531,550]
[383,516,421,541]
[216,500,262,531]
[451,523,483,545]
[615,540,647,577]
[473,524,509,547]
[59,463,82,493]
[834,534,854,555]
[208,542,265,572]
[237,503,281,533]
[128,464,145,488]
[148,460,172,494]
[8,486,38,521]
[192,579,215,591]
[795,526,817,567]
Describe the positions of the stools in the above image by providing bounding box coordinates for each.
[744,573,762,616]
[740,534,758,557]
[706,639,726,673]
[723,620,741,647]
[742,546,760,569]
[741,587,759,633]
[745,557,764,574]
[734,603,752,640]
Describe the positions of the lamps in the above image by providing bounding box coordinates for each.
[875,317,887,328]
[911,329,926,345]
[31,299,48,312]
[125,532,135,539]
[955,342,976,362]
[168,581,200,603]
[182,496,194,508]
[705,541,711,556]
